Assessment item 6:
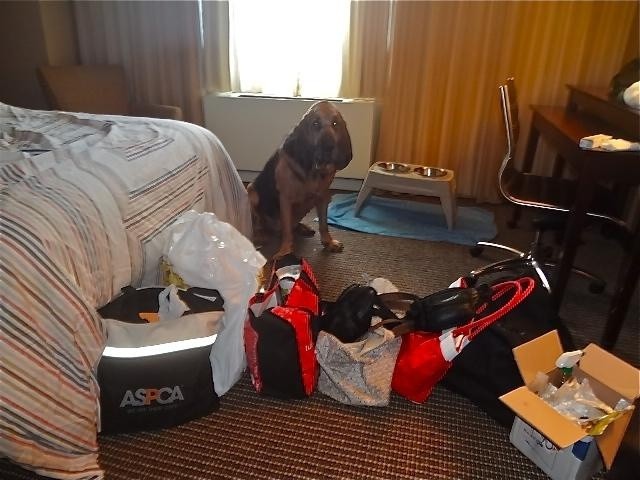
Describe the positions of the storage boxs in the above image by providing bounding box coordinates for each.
[495,328,639,480]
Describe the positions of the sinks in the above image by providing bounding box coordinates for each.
[236,94,346,103]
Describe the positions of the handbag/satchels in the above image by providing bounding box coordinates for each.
[246,252,321,399]
[96,282,225,435]
[164,207,266,398]
[315,251,568,426]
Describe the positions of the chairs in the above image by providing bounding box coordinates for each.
[470,73,625,315]
[37,55,185,124]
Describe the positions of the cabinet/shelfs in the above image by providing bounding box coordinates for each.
[201,89,380,194]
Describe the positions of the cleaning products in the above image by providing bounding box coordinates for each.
[554,349,586,389]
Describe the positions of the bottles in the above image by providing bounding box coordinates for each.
[292,76,302,97]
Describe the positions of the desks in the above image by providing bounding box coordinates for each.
[504,80,640,352]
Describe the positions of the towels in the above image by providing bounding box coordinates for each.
[313,189,499,248]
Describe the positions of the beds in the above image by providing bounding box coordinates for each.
[1,97,255,478]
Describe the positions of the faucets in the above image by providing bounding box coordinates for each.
[293,75,304,98]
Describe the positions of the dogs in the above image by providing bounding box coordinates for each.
[246,101,353,262]
